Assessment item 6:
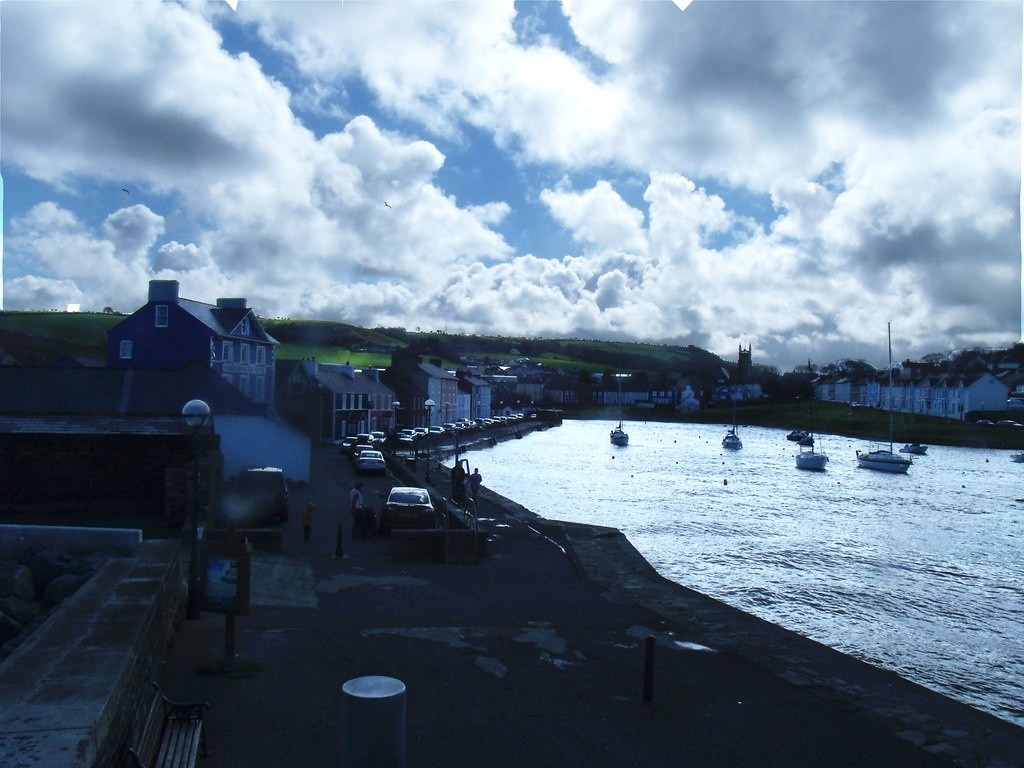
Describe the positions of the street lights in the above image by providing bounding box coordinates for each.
[182,399,210,619]
[393,402,400,456]
[424,399,533,440]
[424,399,435,480]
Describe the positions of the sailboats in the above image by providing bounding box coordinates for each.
[610,371,628,446]
[722,380,742,450]
[795,361,829,470]
[856,322,913,474]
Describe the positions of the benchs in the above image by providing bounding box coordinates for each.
[126,680,212,768]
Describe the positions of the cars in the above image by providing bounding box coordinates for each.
[380,487,436,533]
[355,450,386,476]
[354,444,374,459]
[342,413,536,453]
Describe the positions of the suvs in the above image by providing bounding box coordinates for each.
[238,468,289,524]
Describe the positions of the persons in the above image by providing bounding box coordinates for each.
[346,480,363,540]
[467,468,482,502]
[450,461,466,503]
[302,502,316,543]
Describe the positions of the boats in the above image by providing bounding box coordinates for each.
[786,430,806,441]
[1011,453,1024,463]
[797,436,814,446]
[899,444,928,454]
